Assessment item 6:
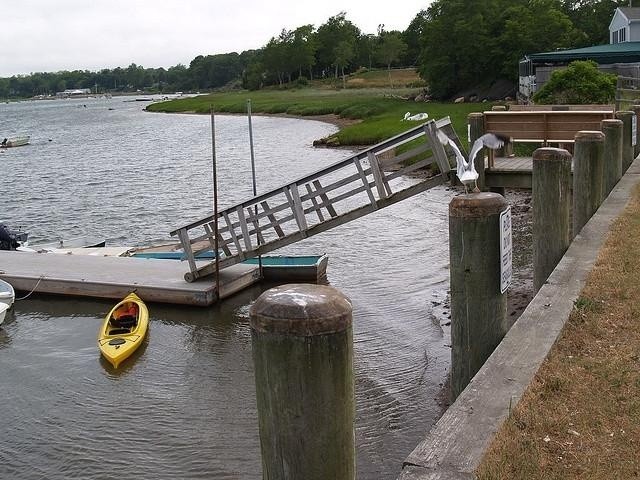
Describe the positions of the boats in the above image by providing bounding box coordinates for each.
[0,135,31,147]
[0,279,16,325]
[0,224,327,283]
[97,292,150,369]
